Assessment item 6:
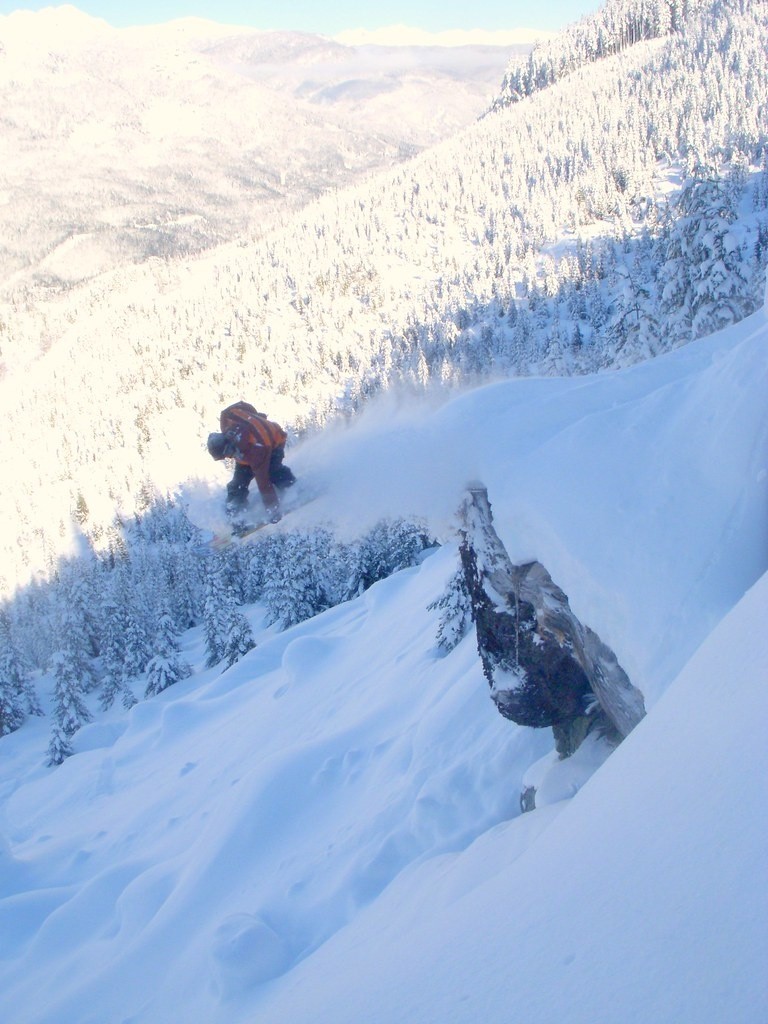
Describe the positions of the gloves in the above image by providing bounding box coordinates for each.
[271,512,282,523]
[258,413,267,419]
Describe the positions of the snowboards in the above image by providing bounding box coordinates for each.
[205,500,312,550]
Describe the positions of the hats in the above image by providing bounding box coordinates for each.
[207,433,236,458]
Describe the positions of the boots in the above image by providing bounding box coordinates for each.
[275,465,297,491]
[225,482,249,516]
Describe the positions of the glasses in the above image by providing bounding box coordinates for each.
[215,445,228,460]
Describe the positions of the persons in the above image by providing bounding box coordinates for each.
[206,401,294,522]
